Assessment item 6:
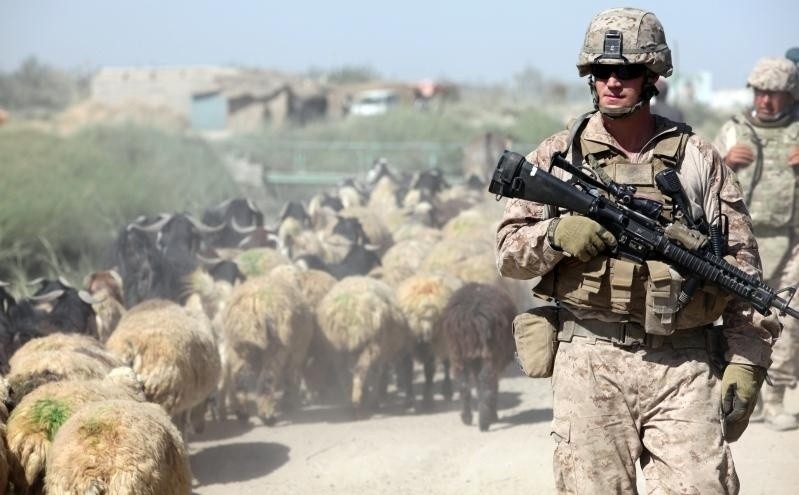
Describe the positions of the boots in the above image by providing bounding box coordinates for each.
[765,386,797,431]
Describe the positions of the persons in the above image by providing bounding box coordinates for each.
[341,91,351,116]
[649,79,685,126]
[786,47,799,68]
[713,55,799,431]
[493,6,783,495]
[680,82,711,128]
[413,88,424,111]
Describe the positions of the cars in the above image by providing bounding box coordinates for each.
[348,86,397,118]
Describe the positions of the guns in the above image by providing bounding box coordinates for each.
[490,151,799,322]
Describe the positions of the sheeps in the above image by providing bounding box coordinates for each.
[0,156,555,495]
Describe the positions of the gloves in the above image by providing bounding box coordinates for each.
[720,362,767,426]
[554,215,618,263]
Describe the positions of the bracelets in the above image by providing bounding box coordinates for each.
[548,217,560,248]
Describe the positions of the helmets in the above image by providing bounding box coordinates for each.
[746,55,798,94]
[575,7,674,76]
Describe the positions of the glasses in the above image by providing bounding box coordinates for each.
[588,64,647,80]
